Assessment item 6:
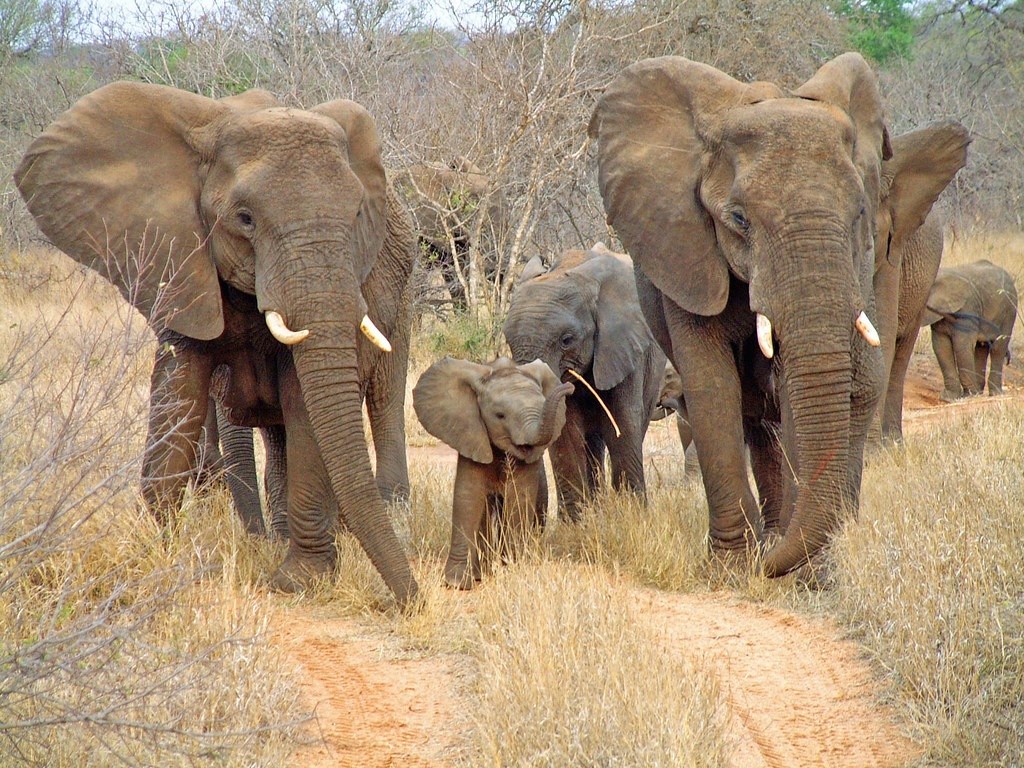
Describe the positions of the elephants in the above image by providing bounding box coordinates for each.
[13,50,1018,618]
